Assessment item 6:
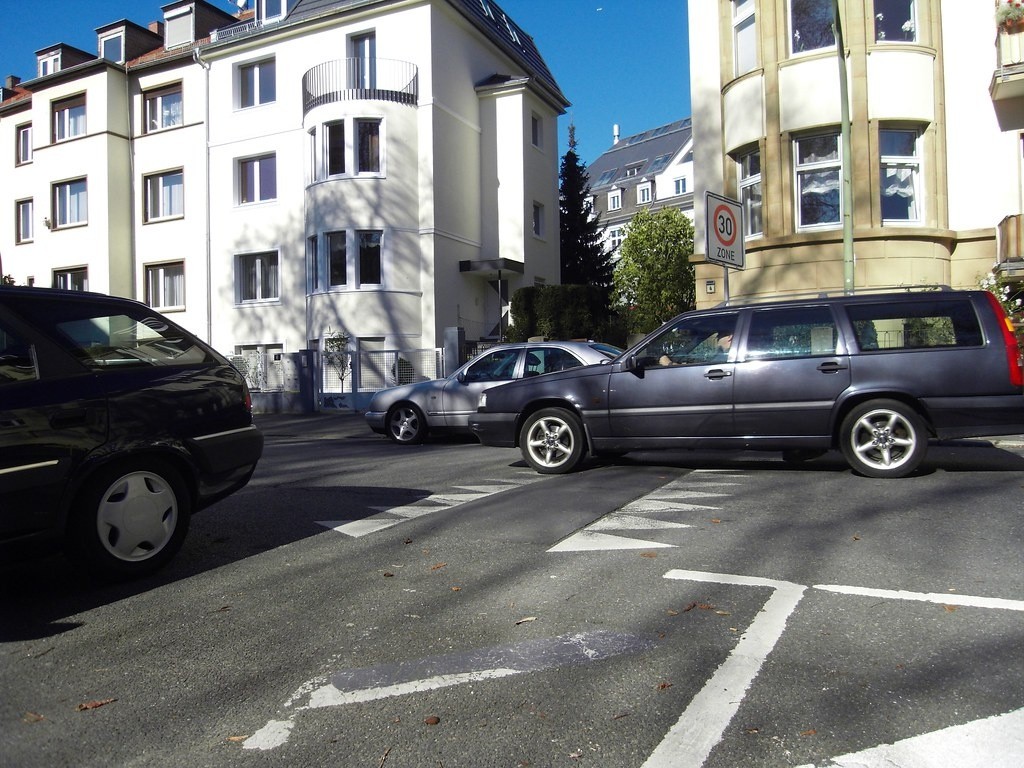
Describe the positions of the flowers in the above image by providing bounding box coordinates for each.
[995,0,1024,26]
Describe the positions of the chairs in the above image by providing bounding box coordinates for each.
[522,353,541,379]
[544,354,561,374]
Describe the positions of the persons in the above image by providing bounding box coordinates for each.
[659,327,735,366]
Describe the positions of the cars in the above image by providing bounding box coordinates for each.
[364,336,630,445]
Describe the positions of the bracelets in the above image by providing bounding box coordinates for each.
[668,361,673,366]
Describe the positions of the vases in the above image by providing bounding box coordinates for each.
[1003,16,1024,30]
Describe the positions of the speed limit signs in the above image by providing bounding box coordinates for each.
[704,190,747,272]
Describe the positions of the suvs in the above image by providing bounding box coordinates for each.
[0,273,266,581]
[467,282,1024,480]
